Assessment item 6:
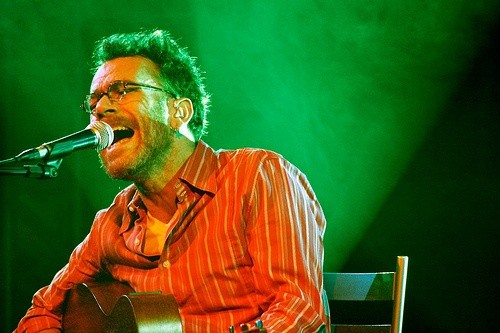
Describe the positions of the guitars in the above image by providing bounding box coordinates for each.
[62,277,271,333]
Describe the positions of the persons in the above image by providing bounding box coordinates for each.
[14,29,331,333]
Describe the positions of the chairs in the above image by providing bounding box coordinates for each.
[323,256,408,333]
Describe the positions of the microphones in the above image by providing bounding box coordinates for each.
[14,120,115,162]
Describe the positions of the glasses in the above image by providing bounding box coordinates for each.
[79,79,176,114]
